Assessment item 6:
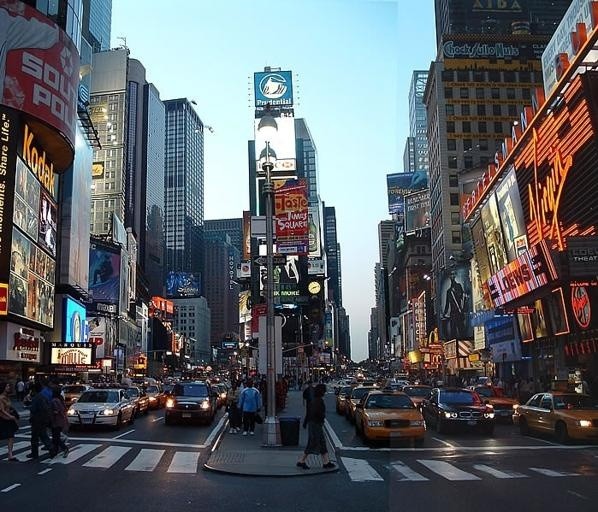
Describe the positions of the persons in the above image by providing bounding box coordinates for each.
[409,375,538,402]
[443,270,468,338]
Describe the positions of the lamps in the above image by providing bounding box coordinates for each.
[82,103,118,149]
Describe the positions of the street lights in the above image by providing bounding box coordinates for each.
[255,100,283,449]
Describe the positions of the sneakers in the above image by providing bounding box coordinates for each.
[296,461,310,469]
[229,427,256,436]
[323,463,335,469]
[7,443,69,462]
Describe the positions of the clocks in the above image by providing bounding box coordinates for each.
[305,275,325,298]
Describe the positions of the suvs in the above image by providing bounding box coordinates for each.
[163,378,217,425]
[419,385,496,437]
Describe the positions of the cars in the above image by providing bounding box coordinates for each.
[509,385,597,444]
[468,383,520,423]
[331,365,438,419]
[63,389,136,428]
[352,386,429,449]
[53,370,230,415]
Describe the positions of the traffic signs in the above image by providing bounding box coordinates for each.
[252,254,287,267]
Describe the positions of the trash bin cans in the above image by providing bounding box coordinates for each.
[279,416,300,446]
